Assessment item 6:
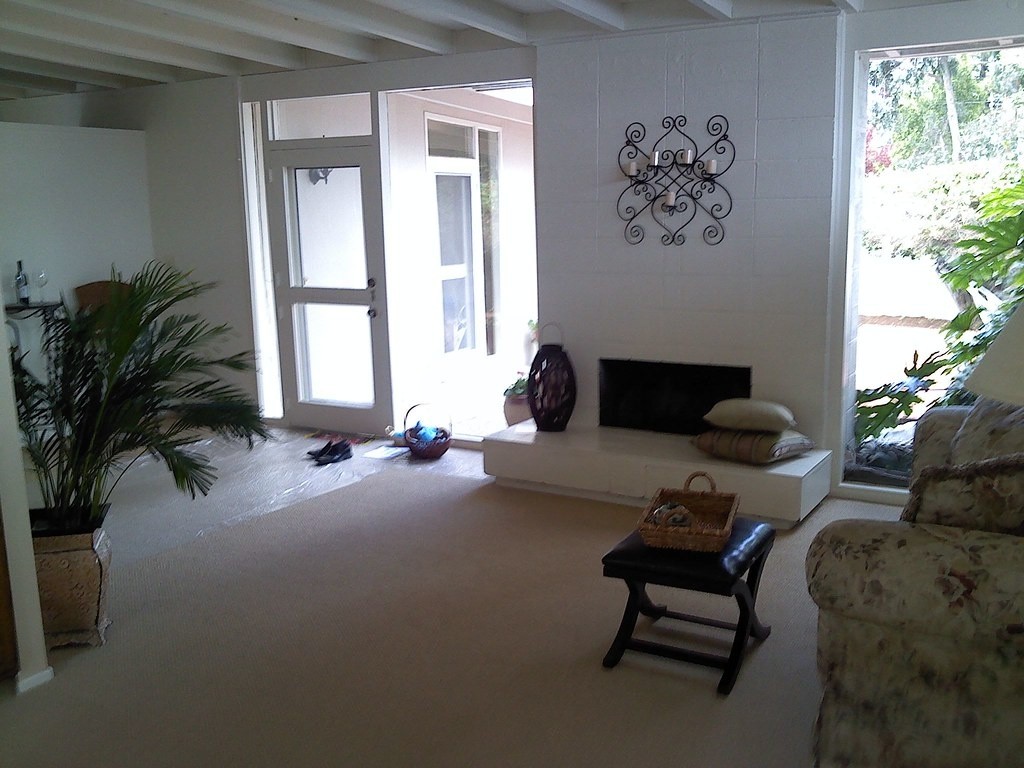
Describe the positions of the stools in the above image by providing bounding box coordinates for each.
[602,518,778,695]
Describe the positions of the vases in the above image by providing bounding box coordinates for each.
[526,344,577,433]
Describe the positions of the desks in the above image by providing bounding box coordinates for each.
[482,416,833,532]
[4,300,64,382]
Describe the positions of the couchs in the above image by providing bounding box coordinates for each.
[805,405,1024,768]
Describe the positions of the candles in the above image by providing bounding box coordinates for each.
[650,151,659,165]
[665,191,675,207]
[706,160,717,174]
[629,161,638,175]
[683,149,694,164]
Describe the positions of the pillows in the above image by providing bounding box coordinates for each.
[688,431,816,466]
[897,395,1024,538]
[704,398,798,432]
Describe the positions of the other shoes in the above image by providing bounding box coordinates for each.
[317,442,353,462]
[307,439,347,455]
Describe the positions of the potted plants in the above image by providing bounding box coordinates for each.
[8,259,279,651]
[502,377,534,427]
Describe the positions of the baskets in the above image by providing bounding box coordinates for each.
[385,425,409,446]
[404,403,453,458]
[637,471,740,553]
